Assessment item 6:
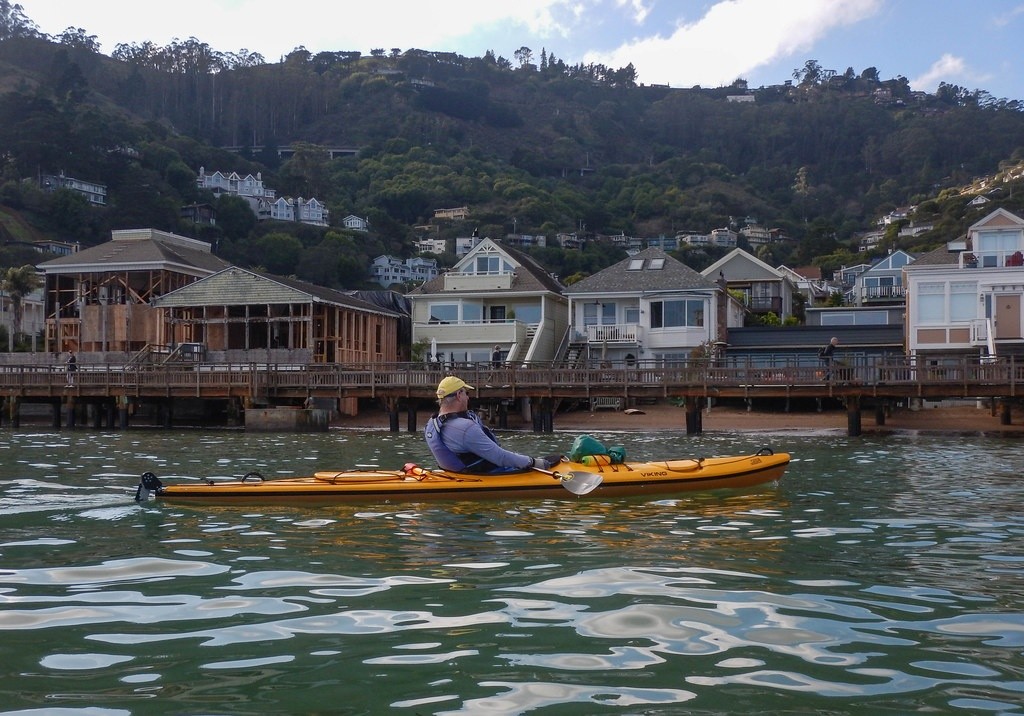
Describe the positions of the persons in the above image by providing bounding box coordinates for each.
[820,337,838,385]
[64,350,76,387]
[486,346,510,387]
[425,375,551,475]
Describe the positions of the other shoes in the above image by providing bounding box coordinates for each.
[502,384,510,387]
[485,384,492,387]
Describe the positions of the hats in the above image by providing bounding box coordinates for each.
[436,376,475,399]
[494,345,501,350]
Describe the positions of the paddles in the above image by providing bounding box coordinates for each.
[532,465,605,496]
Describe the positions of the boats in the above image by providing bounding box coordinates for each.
[134,447,789,506]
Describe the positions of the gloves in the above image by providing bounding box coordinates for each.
[533,458,551,469]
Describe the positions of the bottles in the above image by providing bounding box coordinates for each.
[582,455,610,466]
